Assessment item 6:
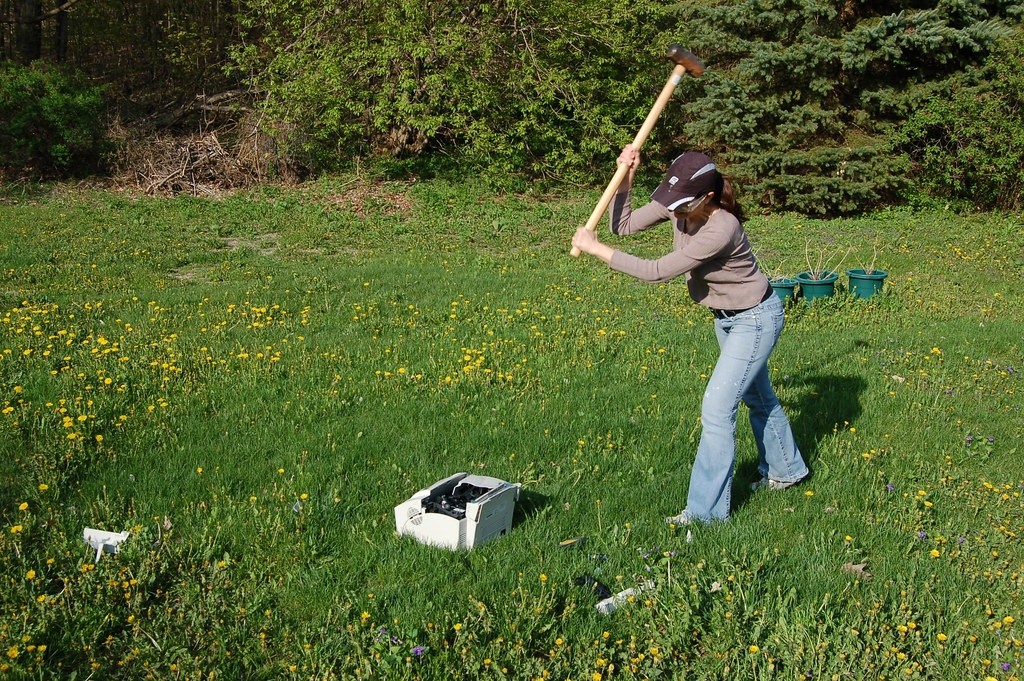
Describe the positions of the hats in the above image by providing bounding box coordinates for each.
[650,152,717,212]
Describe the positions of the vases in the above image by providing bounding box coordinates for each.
[768,277,798,306]
[846,269,888,299]
[796,270,838,300]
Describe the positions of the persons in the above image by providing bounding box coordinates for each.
[572,145,810,523]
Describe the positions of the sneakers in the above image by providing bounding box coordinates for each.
[665,512,691,526]
[748,476,800,492]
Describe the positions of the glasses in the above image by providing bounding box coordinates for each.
[668,193,707,215]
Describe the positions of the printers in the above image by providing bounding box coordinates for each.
[394,473,522,551]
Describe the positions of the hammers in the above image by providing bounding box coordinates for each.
[570,46,705,259]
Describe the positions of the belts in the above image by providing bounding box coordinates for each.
[710,280,773,320]
